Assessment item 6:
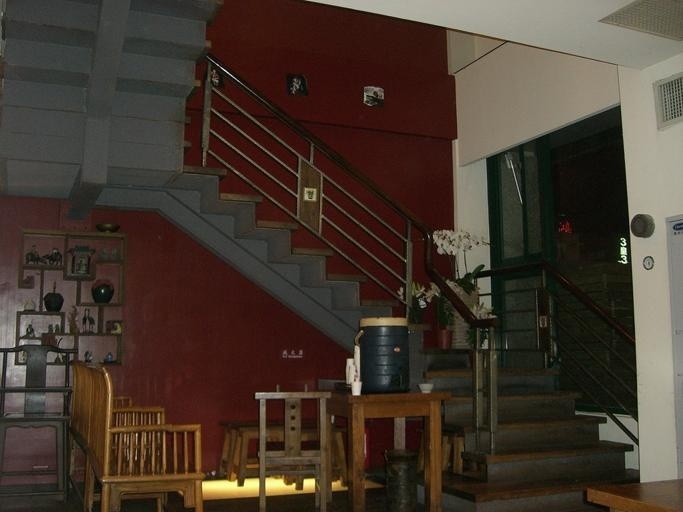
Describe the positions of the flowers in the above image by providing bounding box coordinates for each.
[397,228,492,339]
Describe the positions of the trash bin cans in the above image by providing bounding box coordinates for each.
[386,449,420,512]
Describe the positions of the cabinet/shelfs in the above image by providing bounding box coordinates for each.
[14,228,128,367]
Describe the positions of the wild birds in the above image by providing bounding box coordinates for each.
[82,308,87,333]
[87,309,95,333]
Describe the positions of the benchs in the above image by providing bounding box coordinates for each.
[219,418,348,490]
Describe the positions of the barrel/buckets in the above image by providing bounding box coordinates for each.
[354,316,410,394]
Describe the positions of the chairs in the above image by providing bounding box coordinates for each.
[0,344,78,504]
[255,391,332,512]
[70,362,206,512]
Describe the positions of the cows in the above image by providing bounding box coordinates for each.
[26,252,47,264]
[42,253,62,265]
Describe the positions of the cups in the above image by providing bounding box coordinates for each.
[351,382,363,396]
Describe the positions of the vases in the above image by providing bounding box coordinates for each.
[446,290,479,350]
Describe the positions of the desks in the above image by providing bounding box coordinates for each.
[587,478,683,512]
[326,392,451,512]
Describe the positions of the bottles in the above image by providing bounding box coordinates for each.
[110,245,120,262]
[345,358,355,384]
[99,247,110,263]
[92,283,114,303]
[44,292,64,311]
[106,351,113,363]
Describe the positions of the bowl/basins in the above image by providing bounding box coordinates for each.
[419,383,434,394]
[95,223,120,233]
